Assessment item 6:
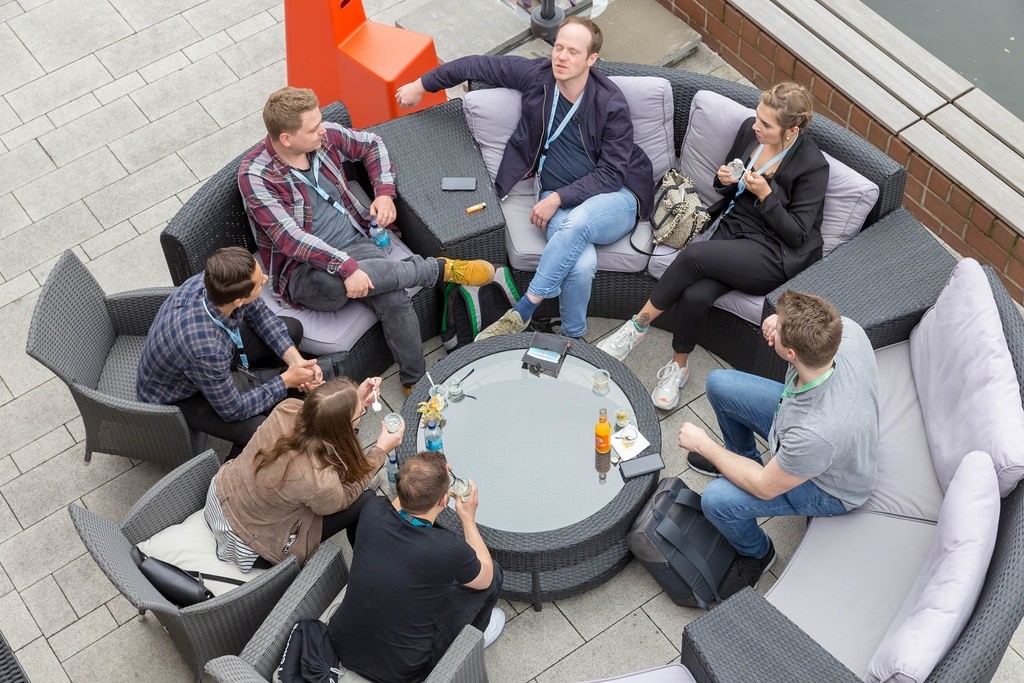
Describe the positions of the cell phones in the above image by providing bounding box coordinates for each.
[619,451,665,478]
[441,177,477,191]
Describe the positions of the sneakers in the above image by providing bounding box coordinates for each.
[715,535,778,600]
[597,320,648,361]
[687,450,765,478]
[404,384,415,397]
[651,359,690,411]
[474,306,533,344]
[436,256,496,287]
[484,606,506,649]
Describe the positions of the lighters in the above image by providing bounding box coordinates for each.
[466,202,487,214]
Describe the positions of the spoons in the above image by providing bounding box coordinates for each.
[615,436,634,441]
[734,158,754,180]
[426,372,443,398]
[451,369,474,389]
[371,378,382,412]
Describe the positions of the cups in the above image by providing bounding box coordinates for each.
[385,413,401,433]
[429,385,449,408]
[447,377,463,398]
[592,370,610,392]
[454,477,473,497]
[728,161,744,179]
[615,408,633,428]
[622,428,638,447]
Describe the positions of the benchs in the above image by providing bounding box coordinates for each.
[462,61,908,373]
[765,258,1024,683]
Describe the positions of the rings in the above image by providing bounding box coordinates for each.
[719,177,722,182]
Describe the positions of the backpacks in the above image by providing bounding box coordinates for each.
[440,264,522,354]
[626,477,732,611]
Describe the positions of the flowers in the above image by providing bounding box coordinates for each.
[417,395,444,428]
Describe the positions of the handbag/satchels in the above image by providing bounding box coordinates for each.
[273,617,340,683]
[131,546,247,606]
[630,170,711,256]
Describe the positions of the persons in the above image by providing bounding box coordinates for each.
[394,19,656,343]
[597,82,830,411]
[237,87,495,398]
[134,247,325,465]
[678,289,882,607]
[328,450,505,683]
[204,376,405,567]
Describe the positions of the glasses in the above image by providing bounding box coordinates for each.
[438,469,456,508]
[352,405,367,424]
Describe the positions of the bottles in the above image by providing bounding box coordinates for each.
[370,220,393,256]
[386,455,399,493]
[424,422,443,454]
[595,408,611,453]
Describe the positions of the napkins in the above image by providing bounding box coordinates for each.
[611,424,651,462]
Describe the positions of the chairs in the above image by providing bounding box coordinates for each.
[204,540,489,683]
[159,101,441,386]
[68,449,301,683]
[26,250,195,467]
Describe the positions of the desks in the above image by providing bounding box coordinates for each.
[681,585,862,683]
[754,207,961,385]
[358,98,507,337]
[395,332,662,612]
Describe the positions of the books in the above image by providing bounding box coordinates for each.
[521,331,570,378]
